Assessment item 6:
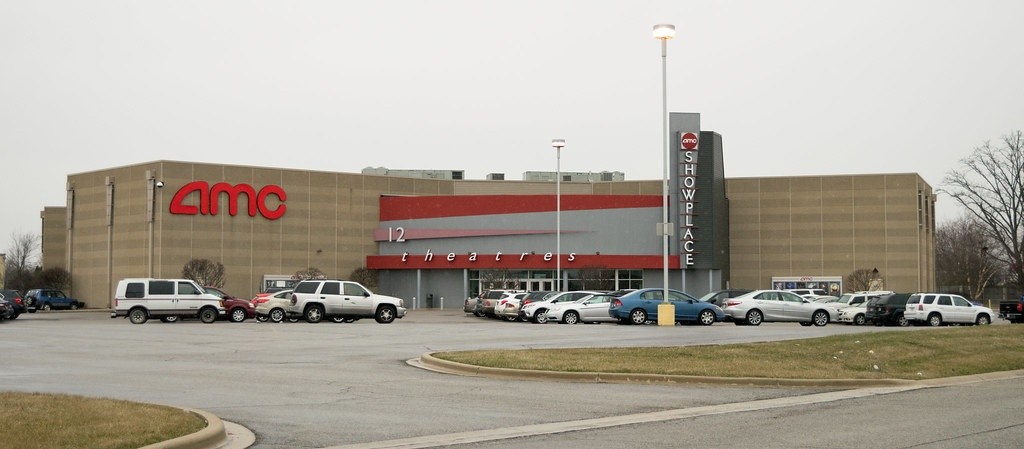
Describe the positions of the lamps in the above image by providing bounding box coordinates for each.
[531,251,535,255]
[468,252,471,255]
[596,251,600,255]
[406,252,409,255]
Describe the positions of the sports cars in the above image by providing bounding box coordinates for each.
[544,294,622,324]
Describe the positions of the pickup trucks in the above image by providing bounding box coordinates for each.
[998,293,1024,323]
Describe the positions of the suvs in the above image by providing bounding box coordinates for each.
[680,289,754,326]
[24,287,81,313]
[904,292,995,326]
[463,288,653,325]
[784,289,915,326]
[288,279,408,323]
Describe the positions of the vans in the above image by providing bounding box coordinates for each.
[110,277,226,324]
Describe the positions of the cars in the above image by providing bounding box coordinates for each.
[721,290,839,326]
[0,290,28,320]
[159,286,356,323]
[609,288,725,324]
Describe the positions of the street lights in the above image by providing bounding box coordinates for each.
[654,23,676,326]
[552,139,566,291]
